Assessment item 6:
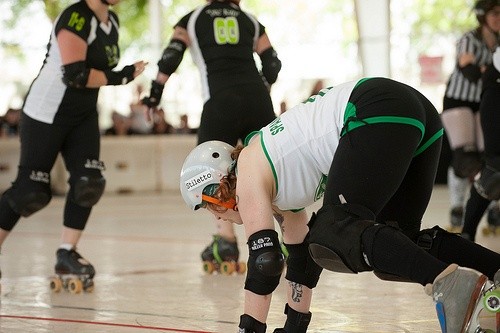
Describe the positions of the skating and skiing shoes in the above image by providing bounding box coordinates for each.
[448,206,466,233]
[49,248,95,294]
[481,205,500,237]
[200,235,245,274]
[424,263,500,333]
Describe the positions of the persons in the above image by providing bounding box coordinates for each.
[180,77,500,332]
[458,44,500,242]
[442,0,500,227]
[139,0,289,274]
[0,1,149,292]
[0,79,327,134]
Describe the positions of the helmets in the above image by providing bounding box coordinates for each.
[179,141,238,211]
[475,1,500,22]
